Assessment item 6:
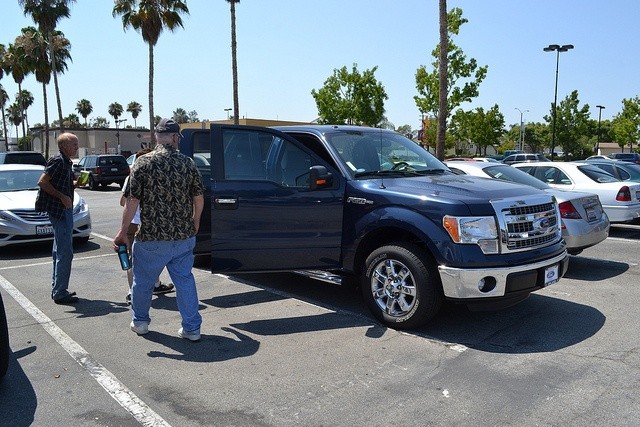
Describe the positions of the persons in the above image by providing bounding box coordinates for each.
[112,120,205,342]
[36,131,87,304]
[120,148,175,305]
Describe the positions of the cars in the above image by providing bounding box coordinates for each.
[510,161,640,224]
[1,163,91,250]
[584,155,613,160]
[438,161,611,256]
[472,157,496,162]
[0,151,47,165]
[579,159,639,183]
[446,157,475,162]
[126,153,138,170]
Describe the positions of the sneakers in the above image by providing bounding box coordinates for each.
[130,320,148,335]
[178,327,201,341]
[125,293,132,305]
[67,290,77,296]
[54,296,79,304]
[153,281,175,294]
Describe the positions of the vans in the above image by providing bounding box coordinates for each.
[504,150,524,158]
[608,153,640,164]
[502,153,552,166]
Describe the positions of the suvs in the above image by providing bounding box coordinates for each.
[73,154,131,190]
[178,122,570,331]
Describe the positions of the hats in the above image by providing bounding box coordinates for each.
[156,119,184,139]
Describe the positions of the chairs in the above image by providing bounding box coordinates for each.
[274,149,305,184]
[340,144,371,170]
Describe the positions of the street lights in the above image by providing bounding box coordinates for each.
[514,106,529,150]
